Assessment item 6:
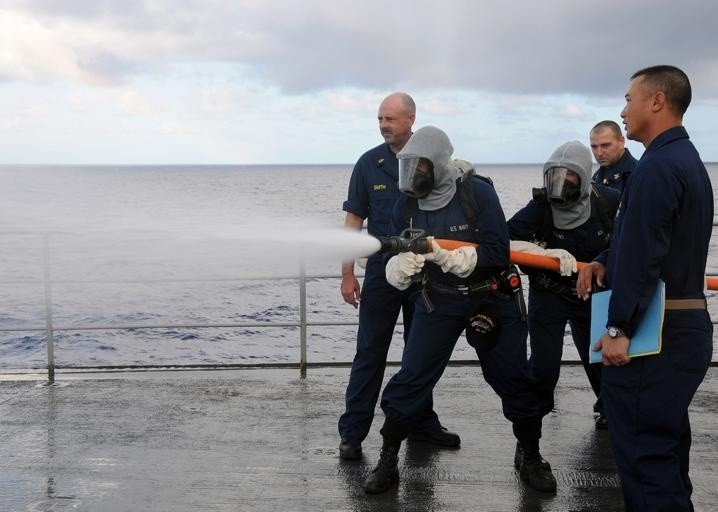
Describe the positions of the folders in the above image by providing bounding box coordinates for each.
[588,279,665,364]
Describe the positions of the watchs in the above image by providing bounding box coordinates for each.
[607,326,624,338]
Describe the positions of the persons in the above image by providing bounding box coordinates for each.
[339,91,461,460]
[506,139,622,473]
[574,64,714,512]
[589,119,638,194]
[359,124,558,494]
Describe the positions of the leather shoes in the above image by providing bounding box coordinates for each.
[406,415,460,447]
[593,399,609,428]
[340,434,362,459]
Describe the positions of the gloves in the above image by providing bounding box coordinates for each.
[384,252,425,291]
[510,239,577,277]
[425,236,478,278]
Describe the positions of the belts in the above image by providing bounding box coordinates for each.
[418,280,498,315]
[663,299,706,311]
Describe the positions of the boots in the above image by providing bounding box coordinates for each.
[514,437,557,492]
[363,443,399,493]
